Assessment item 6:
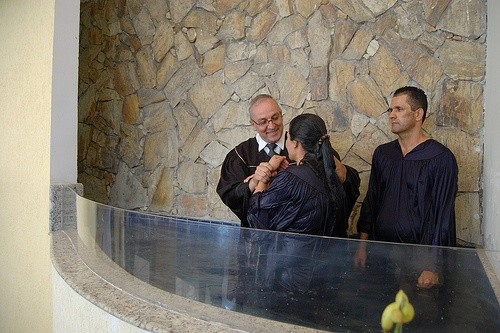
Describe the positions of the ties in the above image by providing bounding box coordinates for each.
[266,143,278,157]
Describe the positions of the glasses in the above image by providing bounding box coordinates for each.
[250,111,281,126]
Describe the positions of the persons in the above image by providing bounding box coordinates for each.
[355,85,459,333]
[246,113,347,326]
[215,94,360,323]
